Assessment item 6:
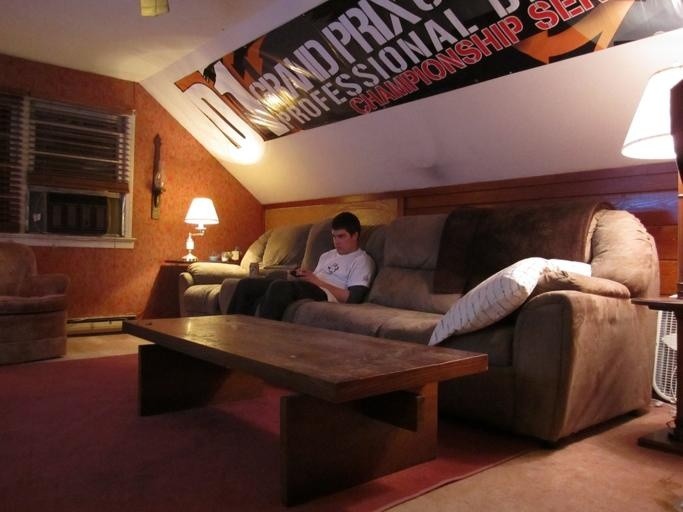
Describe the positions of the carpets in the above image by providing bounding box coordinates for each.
[1,354,536,512]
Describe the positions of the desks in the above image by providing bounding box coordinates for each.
[163,261,241,282]
[632,300,683,456]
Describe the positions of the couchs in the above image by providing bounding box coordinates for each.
[179,203,660,452]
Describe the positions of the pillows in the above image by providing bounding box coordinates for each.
[426,257,592,348]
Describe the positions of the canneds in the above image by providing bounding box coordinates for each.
[249,263,259,277]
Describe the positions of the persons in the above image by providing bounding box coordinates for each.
[226,211,377,322]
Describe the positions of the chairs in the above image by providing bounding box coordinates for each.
[1,241,70,366]
